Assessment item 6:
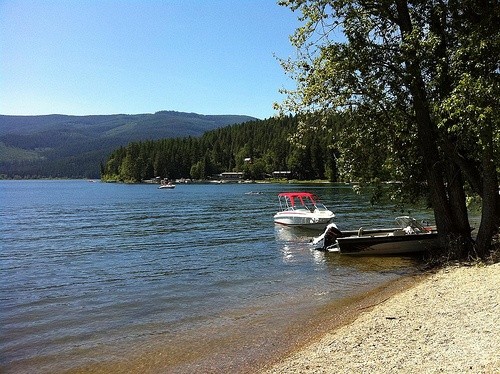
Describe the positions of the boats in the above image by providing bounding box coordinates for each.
[272,192,336,230]
[322,216,439,257]
[158,184,176,189]
